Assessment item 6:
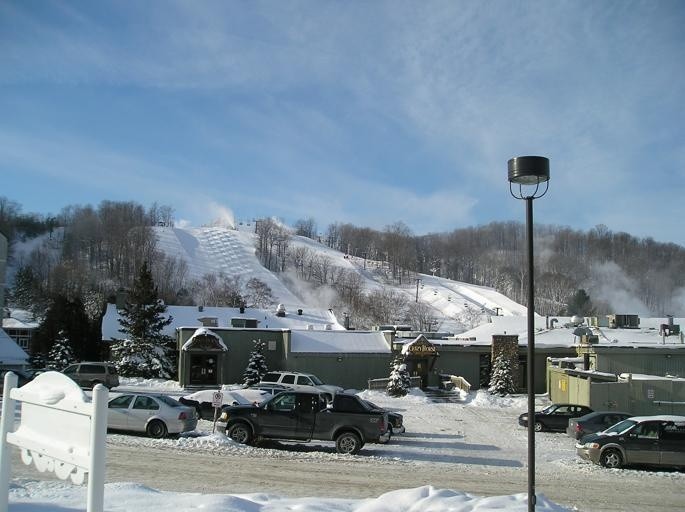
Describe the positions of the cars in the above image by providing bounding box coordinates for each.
[363,399,407,443]
[217,391,391,455]
[516,401,596,432]
[107,391,199,440]
[566,410,638,441]
[0,367,34,397]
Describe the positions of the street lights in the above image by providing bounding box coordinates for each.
[503,154,556,512]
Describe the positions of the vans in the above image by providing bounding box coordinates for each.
[575,414,685,472]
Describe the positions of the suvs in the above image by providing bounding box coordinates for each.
[251,381,334,413]
[258,367,344,404]
[57,359,121,394]
